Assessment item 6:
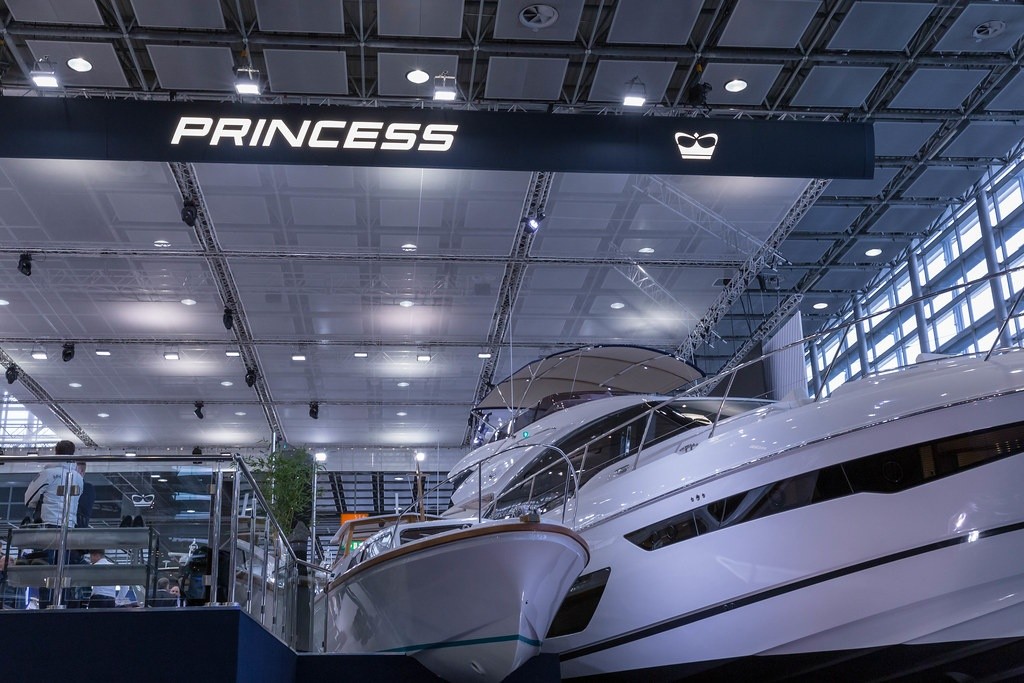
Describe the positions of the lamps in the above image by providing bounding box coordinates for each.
[188,539,198,557]
[623,76,646,107]
[223,306,233,329]
[164,343,180,360]
[31,340,48,359]
[417,347,431,362]
[233,66,261,95]
[62,342,75,362]
[309,401,318,419]
[245,367,256,387]
[192,447,202,464]
[684,83,712,111]
[5,363,19,384]
[17,253,32,276]
[193,401,204,419]
[30,55,60,88]
[433,70,458,101]
[520,205,546,235]
[181,197,197,226]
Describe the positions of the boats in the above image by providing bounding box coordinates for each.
[442,261,1024,683]
[310,510,591,682]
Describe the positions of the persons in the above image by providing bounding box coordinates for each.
[0,441,186,609]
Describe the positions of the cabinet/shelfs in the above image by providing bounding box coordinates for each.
[0,526,161,609]
